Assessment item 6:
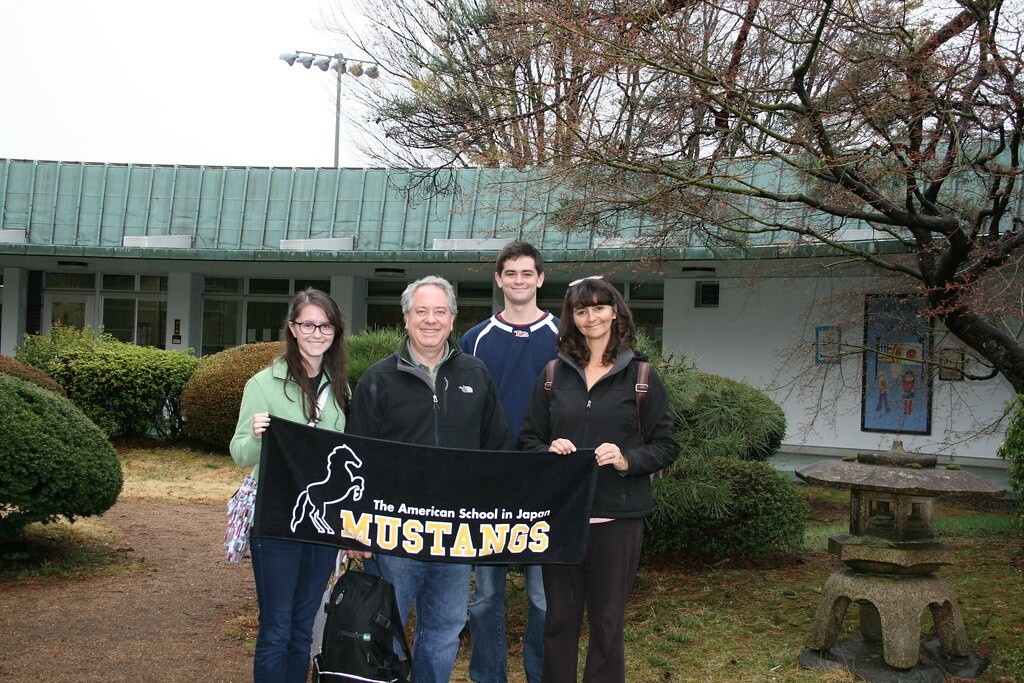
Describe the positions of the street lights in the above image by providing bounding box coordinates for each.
[281,48,378,172]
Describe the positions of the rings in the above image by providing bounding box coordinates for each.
[611,453,615,458]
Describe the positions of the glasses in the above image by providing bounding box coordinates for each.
[289,320,338,336]
[567,274,606,287]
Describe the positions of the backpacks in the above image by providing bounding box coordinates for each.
[313,558,414,683]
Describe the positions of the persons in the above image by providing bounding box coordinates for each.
[228,288,353,683]
[457,240,566,683]
[344,276,514,683]
[516,275,682,682]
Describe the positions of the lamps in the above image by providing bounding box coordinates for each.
[374,268,406,279]
[56,261,88,272]
[278,51,380,79]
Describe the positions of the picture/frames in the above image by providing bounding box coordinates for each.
[938,348,965,382]
[860,293,933,436]
